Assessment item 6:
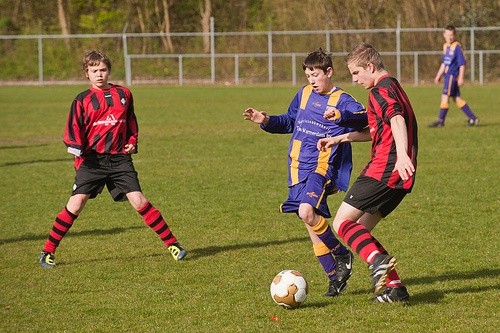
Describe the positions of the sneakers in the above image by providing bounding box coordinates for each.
[371,286,409,303]
[168,243,185,261]
[323,280,347,297]
[40,251,56,268]
[334,248,352,283]
[369,255,397,295]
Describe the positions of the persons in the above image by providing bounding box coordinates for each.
[39,51,187,267]
[243,49,368,296]
[427,25,478,128]
[316,42,418,303]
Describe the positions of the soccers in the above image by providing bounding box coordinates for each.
[268,270,309,308]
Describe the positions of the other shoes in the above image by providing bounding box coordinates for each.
[466,118,478,126]
[429,121,443,128]
